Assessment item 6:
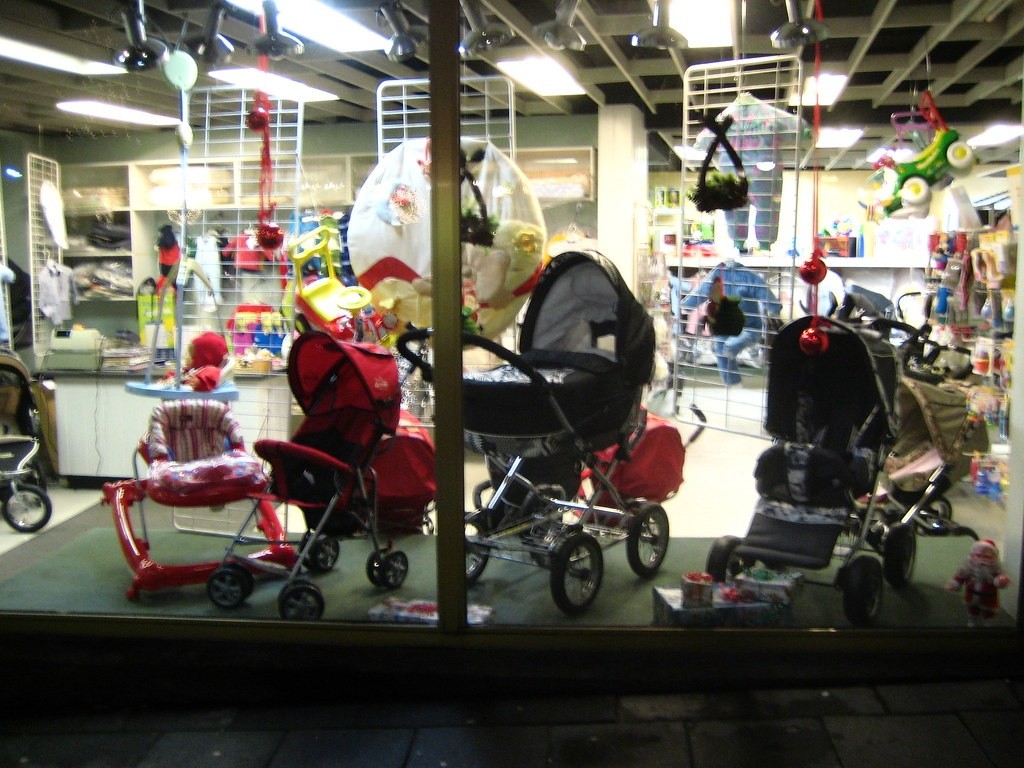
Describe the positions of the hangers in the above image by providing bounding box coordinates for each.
[40,176,256,263]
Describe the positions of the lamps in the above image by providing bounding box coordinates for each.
[459,0,516,60]
[631,0,689,50]
[112,0,170,73]
[245,0,305,61]
[533,0,587,51]
[180,0,235,64]
[376,0,430,61]
[771,0,831,49]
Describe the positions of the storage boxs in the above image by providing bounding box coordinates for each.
[367,596,496,625]
[652,564,804,629]
[817,237,855,257]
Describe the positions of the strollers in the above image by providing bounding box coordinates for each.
[563,385,711,539]
[0,347,57,533]
[204,331,408,624]
[306,410,437,538]
[393,247,672,619]
[700,312,989,625]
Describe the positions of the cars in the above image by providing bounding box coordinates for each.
[856,91,976,222]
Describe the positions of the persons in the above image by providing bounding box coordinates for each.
[139,333,230,393]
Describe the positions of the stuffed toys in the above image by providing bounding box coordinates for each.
[945,539,1012,628]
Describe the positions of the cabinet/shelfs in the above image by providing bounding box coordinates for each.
[58,162,156,303]
[130,145,595,206]
